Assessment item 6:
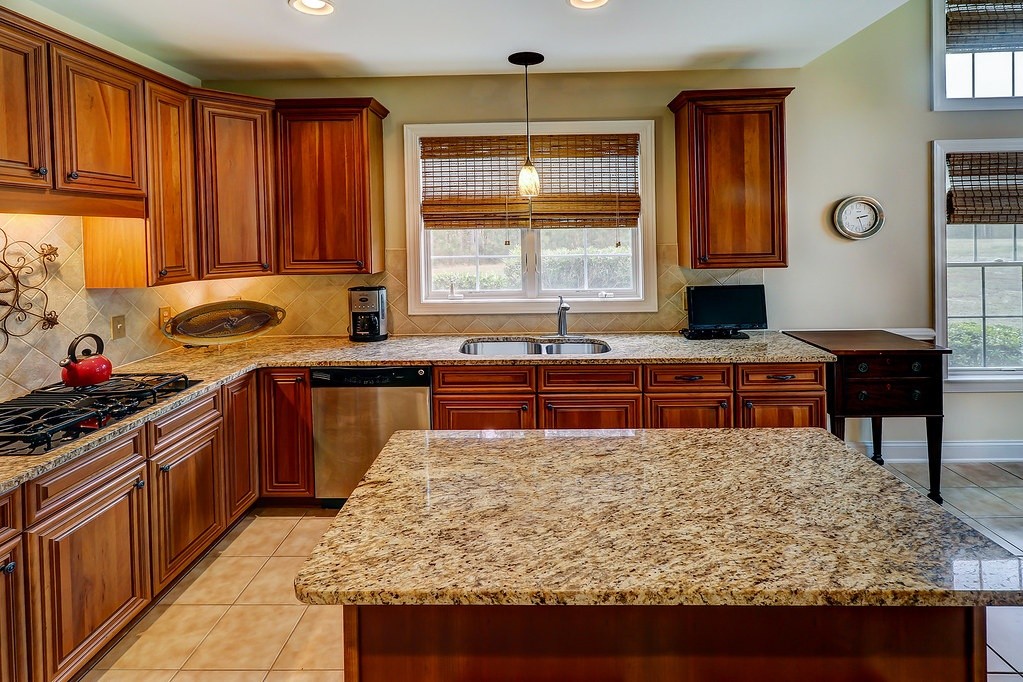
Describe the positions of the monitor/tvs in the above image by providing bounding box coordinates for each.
[687,286,768,338]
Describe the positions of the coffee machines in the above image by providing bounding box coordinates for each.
[347,286,388,342]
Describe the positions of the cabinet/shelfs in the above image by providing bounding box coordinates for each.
[195,83,282,282]
[276,95,390,278]
[665,86,795,270]
[644,362,829,428]
[435,366,643,429]
[83,73,194,294]
[0,369,315,682]
[0,5,147,219]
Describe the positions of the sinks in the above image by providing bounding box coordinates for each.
[545,344,612,354]
[459,342,543,355]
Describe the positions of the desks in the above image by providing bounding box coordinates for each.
[786,328,955,505]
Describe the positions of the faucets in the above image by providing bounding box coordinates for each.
[540,295,585,338]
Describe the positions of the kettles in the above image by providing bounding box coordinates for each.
[59,334,112,387]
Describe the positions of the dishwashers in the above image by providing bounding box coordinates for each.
[309,367,431,509]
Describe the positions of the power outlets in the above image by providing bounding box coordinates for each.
[159,306,173,330]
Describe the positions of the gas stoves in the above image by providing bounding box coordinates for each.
[0,373,199,455]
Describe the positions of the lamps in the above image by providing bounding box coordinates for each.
[507,50,545,196]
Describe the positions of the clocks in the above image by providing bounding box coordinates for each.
[832,196,887,241]
